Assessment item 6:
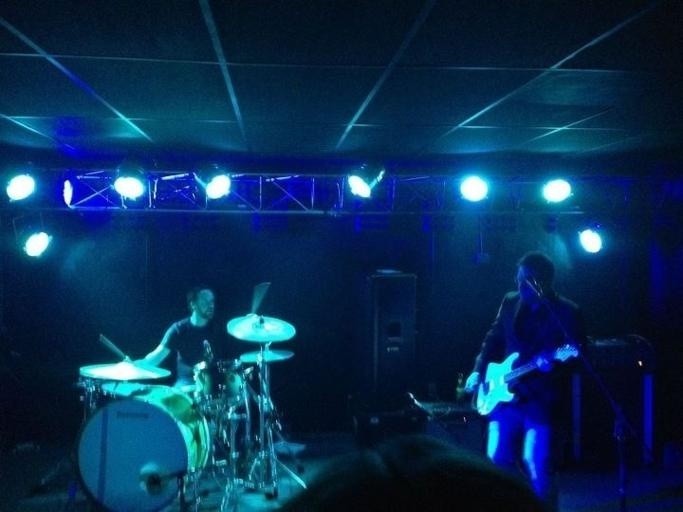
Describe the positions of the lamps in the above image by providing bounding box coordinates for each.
[108,163,150,204]
[191,163,237,202]
[342,165,388,201]
[458,165,605,255]
[1,163,77,258]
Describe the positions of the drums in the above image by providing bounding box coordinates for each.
[75,385,210,512]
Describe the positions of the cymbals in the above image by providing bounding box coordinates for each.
[78,357,171,381]
[239,347,295,364]
[226,313,297,343]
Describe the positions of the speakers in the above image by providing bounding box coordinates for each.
[367,274,417,396]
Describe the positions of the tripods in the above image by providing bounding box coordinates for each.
[193,344,308,511]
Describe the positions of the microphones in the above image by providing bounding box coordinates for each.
[525,275,542,297]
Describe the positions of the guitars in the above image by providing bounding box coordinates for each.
[476,344,579,416]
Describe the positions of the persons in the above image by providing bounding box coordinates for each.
[271,434,549,512]
[464,250,581,500]
[123,280,305,459]
